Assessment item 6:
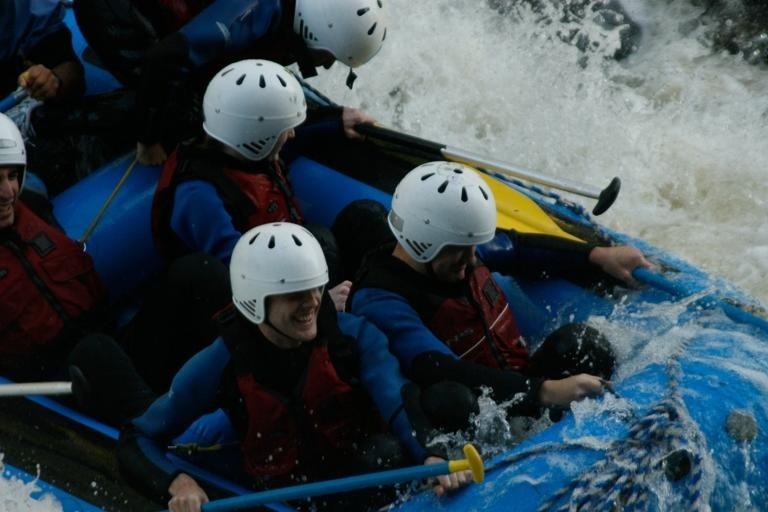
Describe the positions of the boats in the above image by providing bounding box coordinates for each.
[1,83,763,511]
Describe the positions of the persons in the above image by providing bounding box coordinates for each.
[72,1,388,171]
[0,1,100,202]
[148,60,394,288]
[116,226,475,512]
[327,157,656,468]
[0,106,155,434]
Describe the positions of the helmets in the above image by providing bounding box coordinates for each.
[229,223,330,326]
[294,0,388,69]
[386,161,497,264]
[0,87,44,196]
[203,59,308,162]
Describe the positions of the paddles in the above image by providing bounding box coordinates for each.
[454,162,766,331]
